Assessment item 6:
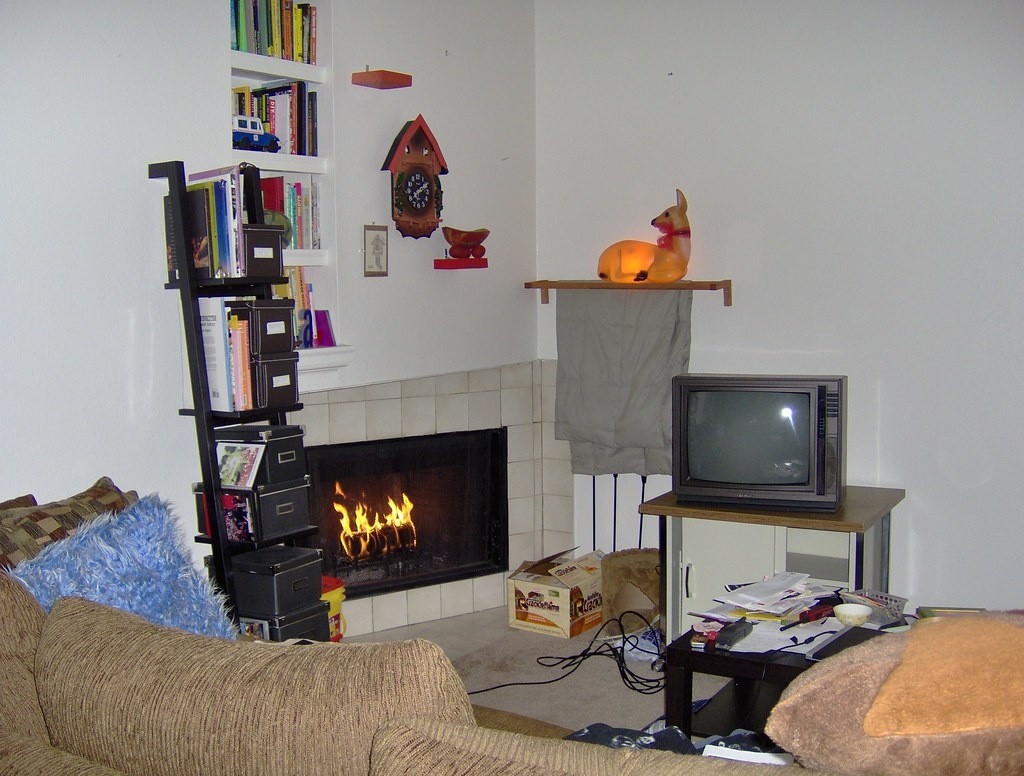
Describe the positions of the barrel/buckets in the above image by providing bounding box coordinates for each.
[321,576,346,642]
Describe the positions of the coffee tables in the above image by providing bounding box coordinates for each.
[658,620,888,750]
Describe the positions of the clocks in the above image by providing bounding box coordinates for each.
[381,116,447,239]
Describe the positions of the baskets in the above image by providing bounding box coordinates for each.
[839,589,909,626]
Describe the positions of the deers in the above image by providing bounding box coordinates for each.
[596,189,691,283]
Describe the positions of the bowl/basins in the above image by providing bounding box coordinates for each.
[834,603,873,627]
[442,227,490,246]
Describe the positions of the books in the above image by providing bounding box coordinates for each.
[195,492,260,541]
[686,570,835,626]
[273,267,338,350]
[164,165,321,282]
[216,443,266,490]
[230,78,318,158]
[198,296,256,412]
[229,0,317,66]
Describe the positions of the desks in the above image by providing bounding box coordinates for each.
[637,485,907,654]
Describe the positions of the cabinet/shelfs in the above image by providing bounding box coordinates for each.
[147,160,332,639]
[230,50,334,267]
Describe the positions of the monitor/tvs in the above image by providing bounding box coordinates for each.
[671,372,848,513]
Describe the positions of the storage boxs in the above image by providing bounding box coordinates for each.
[231,598,329,643]
[506,545,611,641]
[252,350,300,405]
[193,486,311,538]
[204,546,325,617]
[224,297,296,356]
[214,425,308,486]
[243,224,284,272]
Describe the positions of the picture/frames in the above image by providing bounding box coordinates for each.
[917,607,987,618]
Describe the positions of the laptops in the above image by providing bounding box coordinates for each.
[805,625,891,662]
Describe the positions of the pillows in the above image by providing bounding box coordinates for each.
[23,496,238,640]
[0,476,129,572]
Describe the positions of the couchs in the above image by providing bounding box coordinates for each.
[0,490,1023,776]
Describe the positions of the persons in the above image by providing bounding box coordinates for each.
[245,622,262,639]
